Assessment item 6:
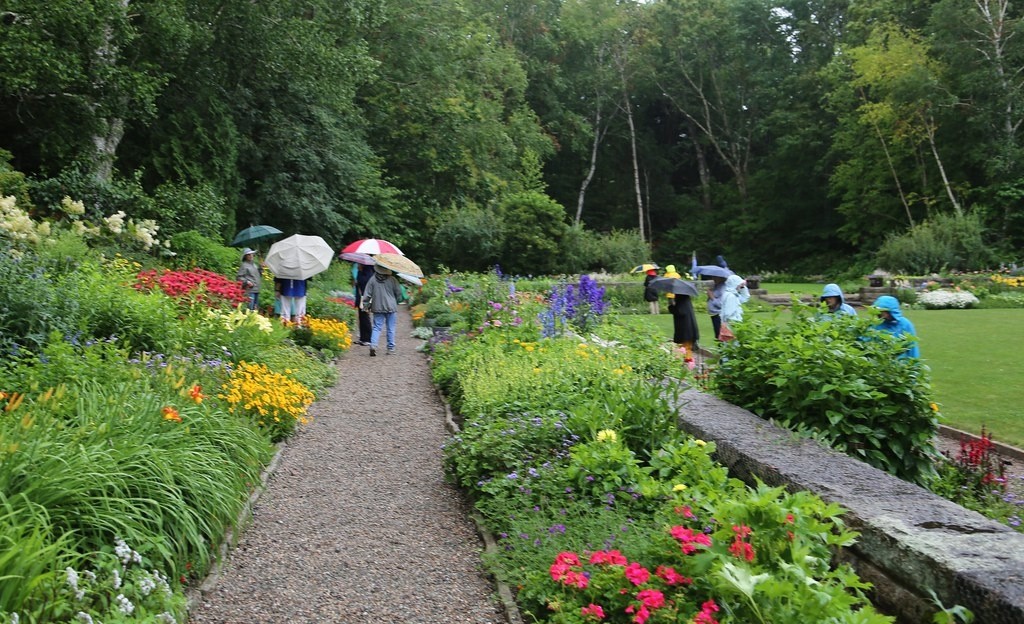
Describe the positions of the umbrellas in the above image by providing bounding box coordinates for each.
[649,277,699,297]
[338,253,425,287]
[265,232,336,280]
[340,237,405,256]
[231,225,284,247]
[630,264,661,274]
[698,266,735,280]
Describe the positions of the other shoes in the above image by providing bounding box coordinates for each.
[387,349,396,354]
[353,340,370,346]
[369,348,375,357]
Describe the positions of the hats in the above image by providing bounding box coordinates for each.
[374,263,393,275]
[241,248,256,262]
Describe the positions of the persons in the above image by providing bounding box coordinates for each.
[664,263,683,305]
[255,251,266,274]
[644,276,661,314]
[668,291,700,351]
[718,274,751,371]
[272,276,312,324]
[811,284,858,345]
[236,247,260,311]
[352,262,375,348]
[859,293,920,366]
[705,277,727,340]
[360,266,397,355]
